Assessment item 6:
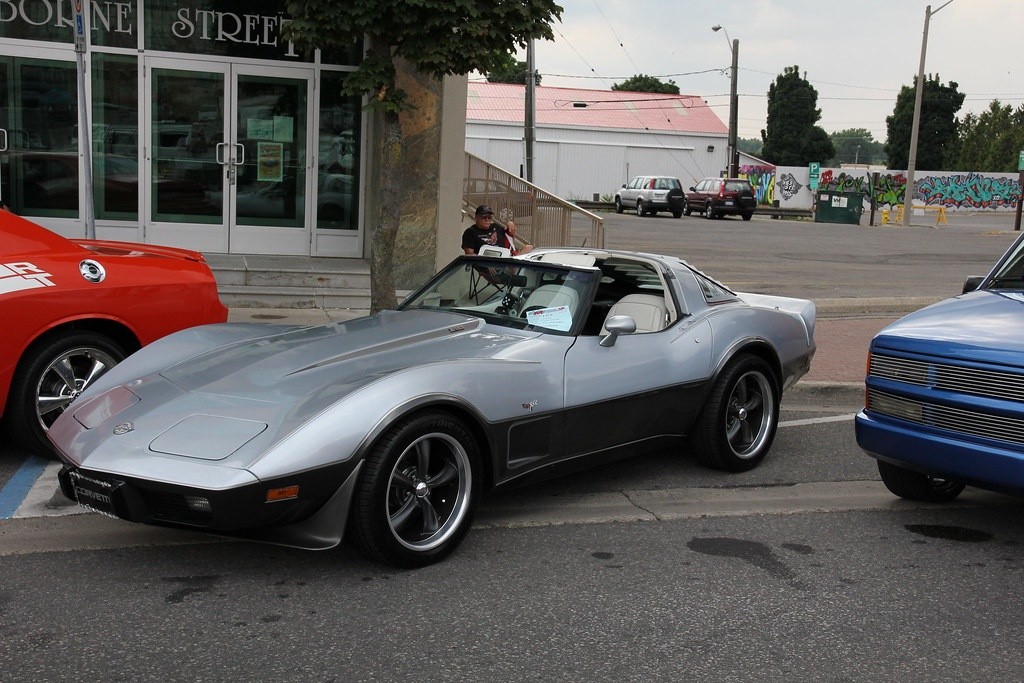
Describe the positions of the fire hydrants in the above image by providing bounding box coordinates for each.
[881,207,891,225]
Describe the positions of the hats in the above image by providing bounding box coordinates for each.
[475,205,494,215]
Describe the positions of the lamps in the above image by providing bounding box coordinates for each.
[708,145,714,152]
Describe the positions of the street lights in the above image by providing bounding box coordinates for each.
[711,24,739,179]
[855,144,861,169]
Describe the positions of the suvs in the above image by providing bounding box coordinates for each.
[614,176,687,219]
[853,230,1024,504]
[681,178,757,220]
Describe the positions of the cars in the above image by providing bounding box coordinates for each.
[0,206,228,459]
[0,122,359,230]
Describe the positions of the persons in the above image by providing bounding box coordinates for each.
[176,122,227,153]
[462,204,534,285]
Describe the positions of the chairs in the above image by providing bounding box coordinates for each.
[600,295,667,335]
[518,285,579,332]
[465,232,519,306]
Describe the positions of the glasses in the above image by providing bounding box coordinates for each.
[479,216,492,220]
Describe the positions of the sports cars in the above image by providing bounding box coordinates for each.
[47,246,817,570]
